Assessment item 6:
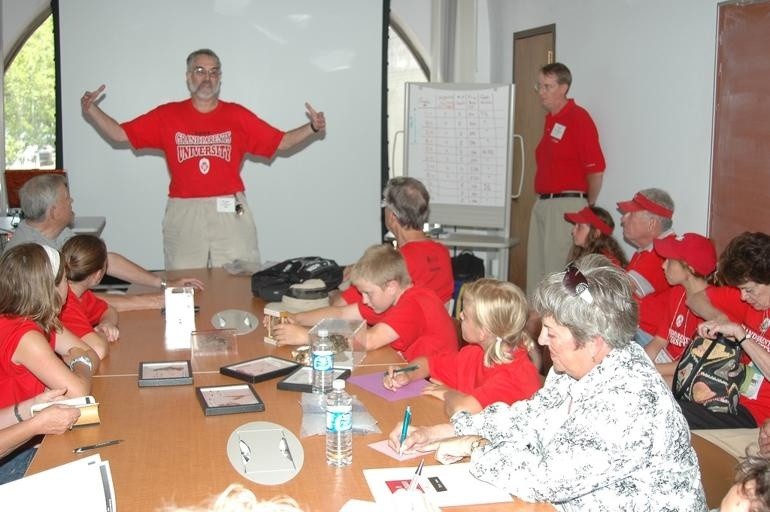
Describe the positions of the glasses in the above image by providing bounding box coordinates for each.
[534,82,557,92]
[564,259,597,306]
[189,65,222,79]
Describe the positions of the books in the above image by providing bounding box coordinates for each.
[29,395,100,430]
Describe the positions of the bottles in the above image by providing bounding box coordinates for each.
[310,330,335,397]
[326,378,355,467]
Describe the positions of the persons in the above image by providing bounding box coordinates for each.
[81,48,326,269]
[713,459,770,511]
[0,172,205,312]
[59,233,120,360]
[386,253,709,512]
[526,62,606,304]
[0,385,80,459]
[0,244,98,483]
[264,177,770,430]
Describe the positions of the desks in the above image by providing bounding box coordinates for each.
[0,216,106,235]
[22,268,558,512]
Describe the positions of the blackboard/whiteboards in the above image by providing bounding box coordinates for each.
[404,82,516,228]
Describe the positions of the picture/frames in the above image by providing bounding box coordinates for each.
[138,360,193,387]
[277,364,351,394]
[195,384,265,416]
[220,354,302,384]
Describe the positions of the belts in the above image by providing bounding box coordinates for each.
[538,190,590,201]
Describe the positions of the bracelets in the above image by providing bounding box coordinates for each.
[161,278,167,290]
[14,401,24,424]
[310,123,318,133]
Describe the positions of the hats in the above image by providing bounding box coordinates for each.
[564,207,614,235]
[616,192,674,219]
[653,231,717,276]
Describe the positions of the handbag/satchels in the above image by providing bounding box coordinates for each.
[449,247,486,281]
[251,256,346,300]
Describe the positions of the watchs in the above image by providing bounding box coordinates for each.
[70,355,93,372]
[467,435,487,453]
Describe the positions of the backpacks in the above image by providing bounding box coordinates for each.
[672,330,749,416]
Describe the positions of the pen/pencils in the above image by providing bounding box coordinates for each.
[407,458,425,491]
[383,364,420,376]
[71,440,124,455]
[400,405,413,455]
